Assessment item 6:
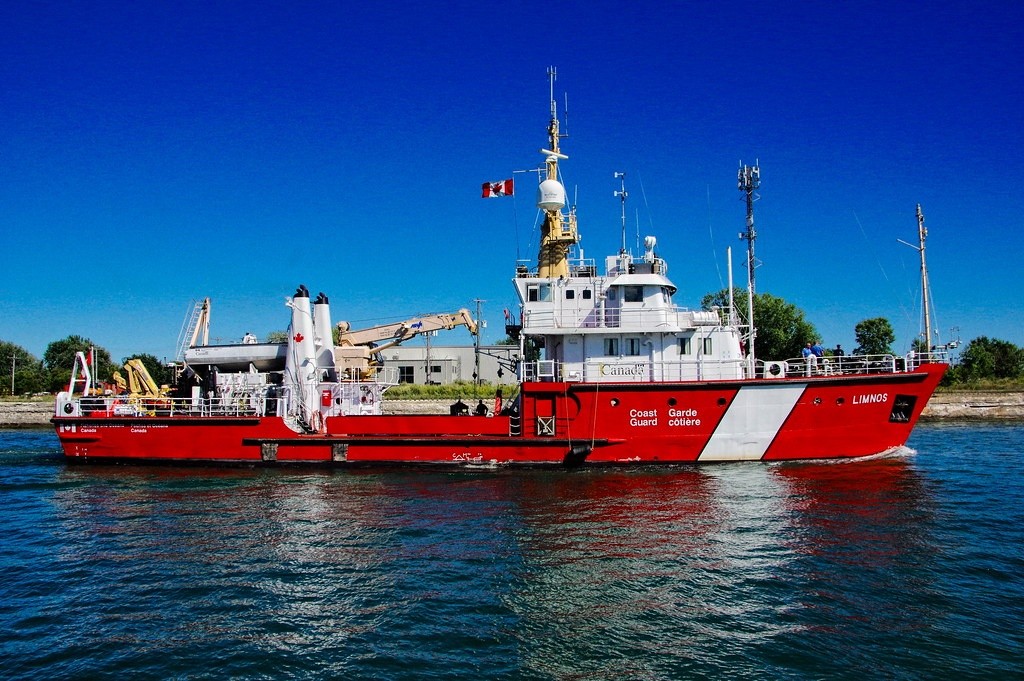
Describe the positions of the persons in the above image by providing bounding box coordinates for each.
[475,400,488,414]
[834,345,845,368]
[810,340,824,376]
[802,343,812,376]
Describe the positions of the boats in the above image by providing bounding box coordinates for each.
[49,65,951,465]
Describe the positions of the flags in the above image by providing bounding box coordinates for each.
[77,350,92,385]
[481,179,513,198]
